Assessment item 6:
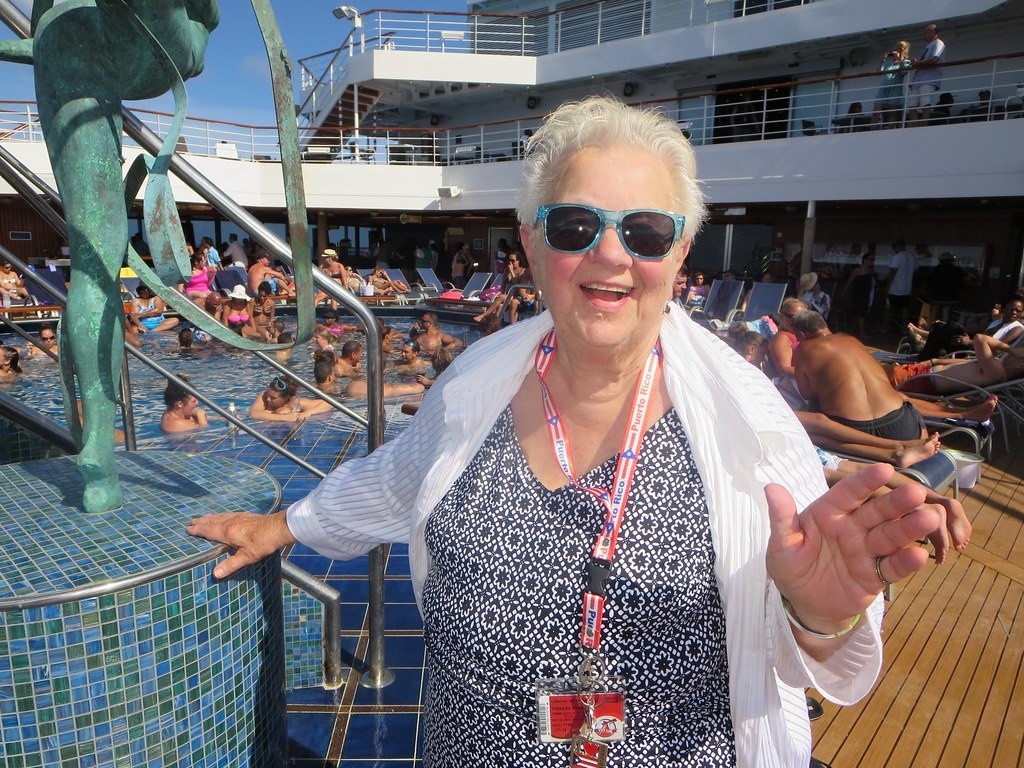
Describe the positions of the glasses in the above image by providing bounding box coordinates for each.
[378,270,384,274]
[866,258,876,261]
[347,269,352,272]
[40,335,55,340]
[782,312,795,321]
[271,375,289,393]
[419,318,434,324]
[508,258,519,264]
[266,257,271,261]
[537,203,686,263]
[403,340,420,351]
[4,266,12,269]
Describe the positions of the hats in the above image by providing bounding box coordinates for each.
[228,285,251,302]
[798,272,818,291]
[136,280,155,298]
[257,252,270,259]
[321,310,336,318]
[321,249,337,257]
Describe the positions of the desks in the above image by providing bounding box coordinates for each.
[964,103,1023,121]
[831,115,872,133]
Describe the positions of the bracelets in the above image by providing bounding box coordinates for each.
[930,359,934,366]
[781,604,861,640]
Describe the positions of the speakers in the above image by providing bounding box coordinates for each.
[438,186,458,197]
[332,5,350,19]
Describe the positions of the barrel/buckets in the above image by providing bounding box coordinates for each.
[363,286,374,296]
[944,450,985,489]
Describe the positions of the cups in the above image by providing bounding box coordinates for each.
[416,368,426,383]
[288,397,301,413]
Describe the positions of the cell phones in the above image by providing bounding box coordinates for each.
[887,53,893,56]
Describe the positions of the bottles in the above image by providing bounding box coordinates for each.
[227,403,238,431]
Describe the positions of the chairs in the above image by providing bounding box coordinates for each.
[20,260,1023,541]
[800,119,837,137]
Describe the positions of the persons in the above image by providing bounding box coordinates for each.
[1,227,1024,567]
[187,22,1024,768]
[0,0,221,513]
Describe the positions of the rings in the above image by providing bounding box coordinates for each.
[876,557,890,585]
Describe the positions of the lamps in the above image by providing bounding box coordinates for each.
[332,6,362,28]
[438,186,462,198]
